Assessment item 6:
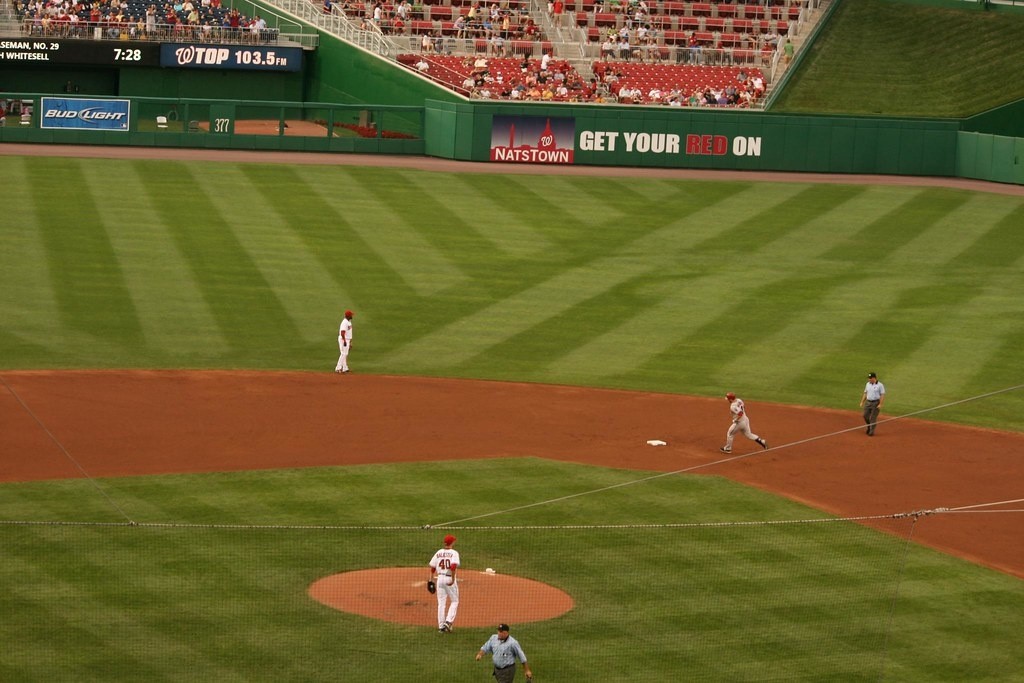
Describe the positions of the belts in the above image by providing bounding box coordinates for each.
[867,399,879,402]
[496,663,515,670]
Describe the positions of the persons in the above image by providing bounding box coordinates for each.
[860,372,885,436]
[720,392,768,454]
[13,0,267,44]
[321,0,796,107]
[334,310,354,373]
[64,79,71,92]
[427,535,460,632]
[476,624,532,683]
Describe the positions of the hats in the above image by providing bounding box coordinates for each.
[866,372,876,378]
[725,392,735,400]
[497,624,509,631]
[444,535,457,546]
[344,310,354,317]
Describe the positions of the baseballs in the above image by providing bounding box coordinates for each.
[430,585,433,588]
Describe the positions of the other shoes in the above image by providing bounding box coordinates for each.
[334,369,353,374]
[866,428,875,436]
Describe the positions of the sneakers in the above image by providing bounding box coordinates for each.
[443,621,453,633]
[438,628,449,633]
[761,439,768,452]
[719,446,732,454]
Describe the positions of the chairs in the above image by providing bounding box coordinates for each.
[322,0,802,109]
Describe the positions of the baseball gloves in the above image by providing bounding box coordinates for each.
[427,581,436,594]
